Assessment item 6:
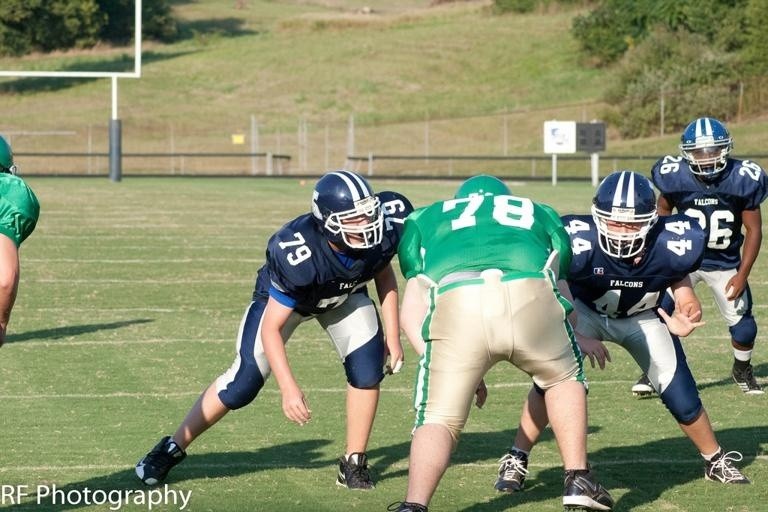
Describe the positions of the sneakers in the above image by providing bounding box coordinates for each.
[493,449,528,490]
[631,376,655,396]
[563,463,613,510]
[136,436,186,486]
[704,447,749,484]
[336,452,375,490]
[733,363,764,395]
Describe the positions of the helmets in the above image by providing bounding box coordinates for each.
[591,173,656,259]
[0,136,18,174]
[453,175,511,198]
[679,118,731,177]
[312,171,383,249]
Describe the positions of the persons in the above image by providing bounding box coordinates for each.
[0,136,40,346]
[496,169,750,493]
[630,117,768,395]
[135,170,415,490]
[388,174,616,512]
